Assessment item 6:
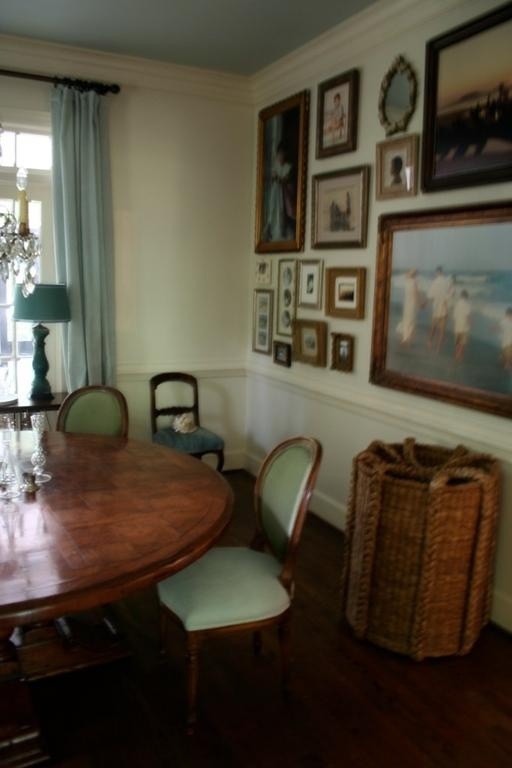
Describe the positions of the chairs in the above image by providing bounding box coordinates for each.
[56,386,128,437]
[152,435,323,735]
[151,373,224,473]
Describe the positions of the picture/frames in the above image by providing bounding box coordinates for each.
[316,68,358,160]
[376,137,419,201]
[252,258,365,372]
[311,163,370,249]
[254,88,309,254]
[368,199,512,419]
[421,4,512,192]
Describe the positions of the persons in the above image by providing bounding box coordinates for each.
[453,290,472,360]
[396,267,425,346]
[327,94,345,142]
[427,266,454,355]
[489,306,511,371]
[390,157,403,186]
[264,145,295,240]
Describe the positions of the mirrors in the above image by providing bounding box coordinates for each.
[378,54,417,137]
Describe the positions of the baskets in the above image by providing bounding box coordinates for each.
[346,438,501,661]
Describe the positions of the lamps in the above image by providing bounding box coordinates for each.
[15,284,71,400]
[0,166,44,298]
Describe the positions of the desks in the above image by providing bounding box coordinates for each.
[0,390,69,430]
